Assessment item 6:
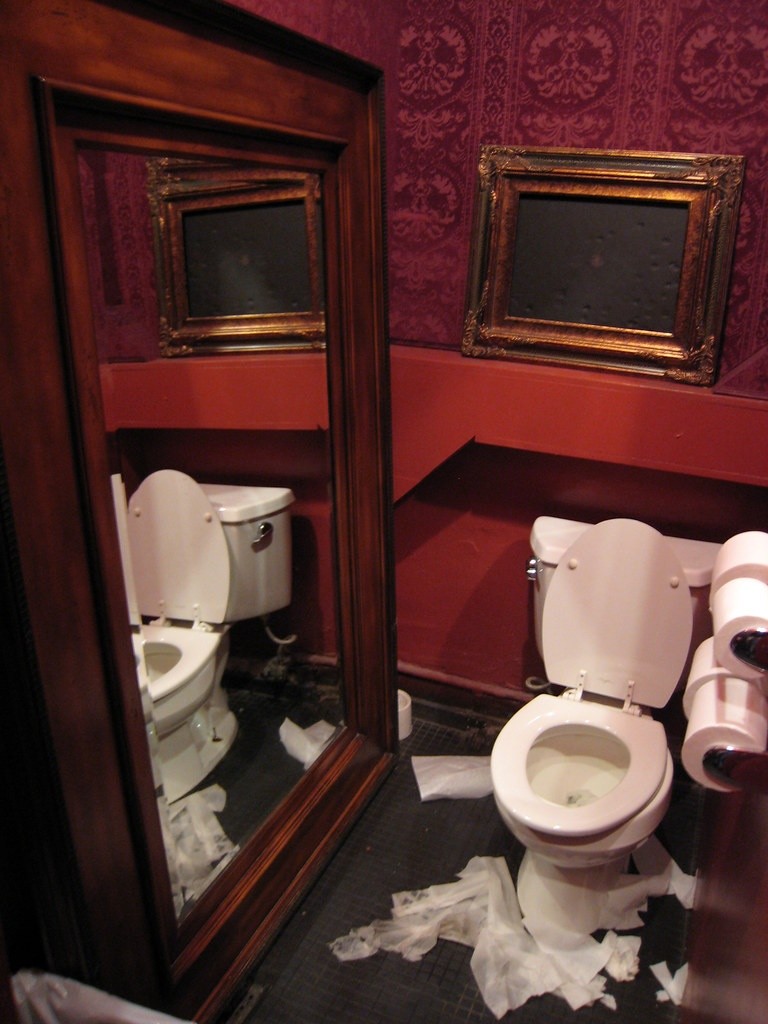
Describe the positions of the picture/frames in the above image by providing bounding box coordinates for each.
[146,150,327,358]
[458,144,746,387]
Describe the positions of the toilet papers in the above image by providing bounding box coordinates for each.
[679,530,768,794]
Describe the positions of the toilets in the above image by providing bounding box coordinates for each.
[489,515,726,952]
[126,468,297,807]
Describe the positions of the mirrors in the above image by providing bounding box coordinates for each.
[0,0,398,1024]
